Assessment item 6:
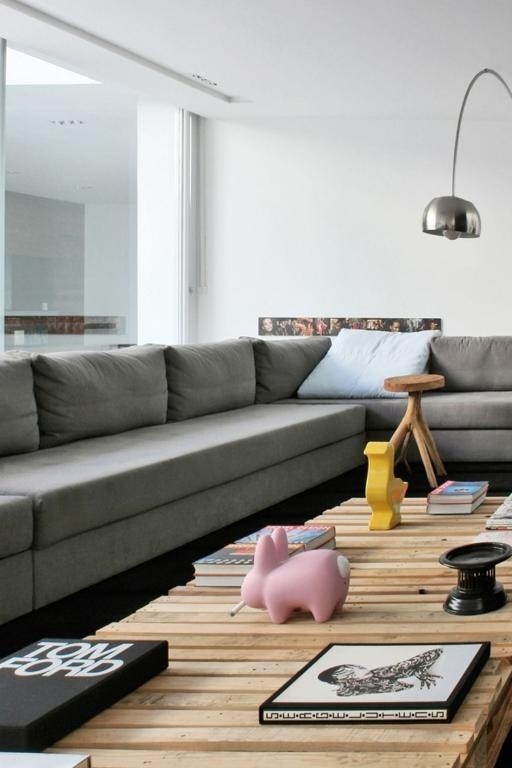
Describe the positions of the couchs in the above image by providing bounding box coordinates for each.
[369,329,512,472]
[1,319,369,625]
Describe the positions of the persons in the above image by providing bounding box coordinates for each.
[318,649,443,696]
[263,318,440,336]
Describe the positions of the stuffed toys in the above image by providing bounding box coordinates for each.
[241,529,352,622]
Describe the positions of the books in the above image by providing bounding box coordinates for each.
[259,643,491,723]
[193,523,336,587]
[0,638,169,768]
[424,481,512,529]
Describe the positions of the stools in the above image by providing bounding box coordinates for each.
[381,372,447,495]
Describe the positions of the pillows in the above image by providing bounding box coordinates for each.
[296,328,442,400]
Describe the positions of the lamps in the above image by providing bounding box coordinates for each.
[419,67,510,245]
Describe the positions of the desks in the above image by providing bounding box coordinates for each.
[47,480,511,768]
